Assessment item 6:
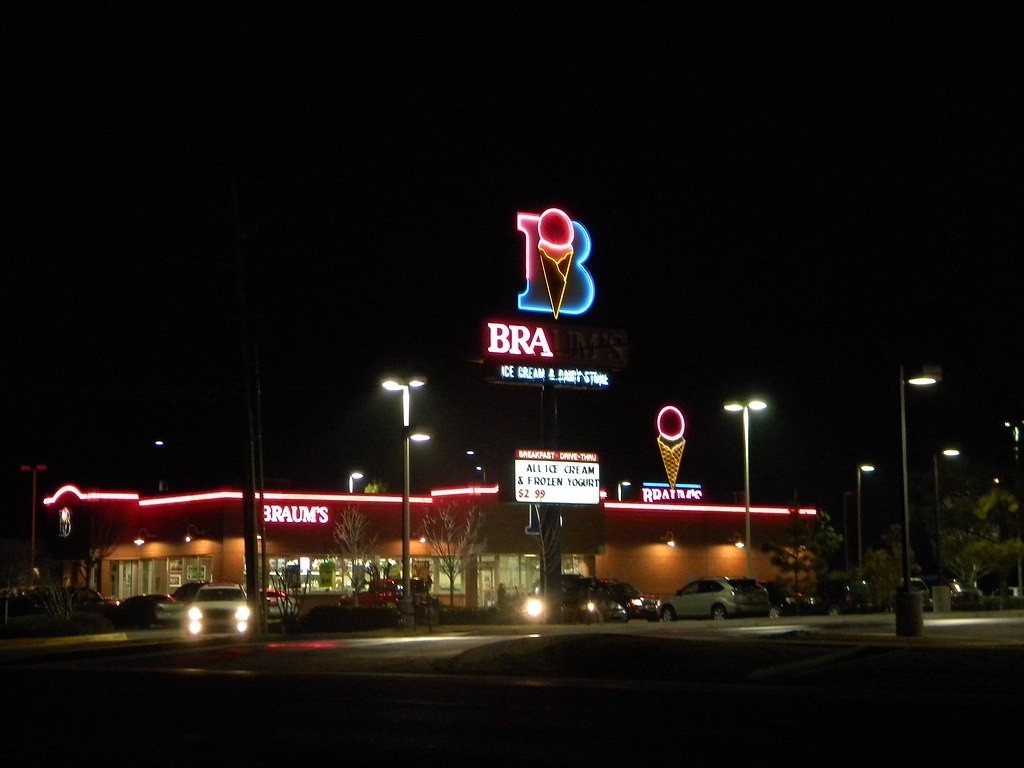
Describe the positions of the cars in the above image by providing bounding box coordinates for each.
[512,575,661,626]
[184,580,253,633]
[656,575,787,623]
[900,577,932,610]
[51,583,294,631]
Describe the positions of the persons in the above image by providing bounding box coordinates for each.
[496,582,507,605]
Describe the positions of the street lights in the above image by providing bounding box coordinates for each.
[842,489,852,591]
[381,375,431,631]
[930,440,963,612]
[18,461,48,589]
[891,360,941,641]
[476,465,487,485]
[723,395,767,577]
[348,469,364,494]
[855,463,876,580]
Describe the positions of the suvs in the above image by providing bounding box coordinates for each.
[344,575,425,610]
